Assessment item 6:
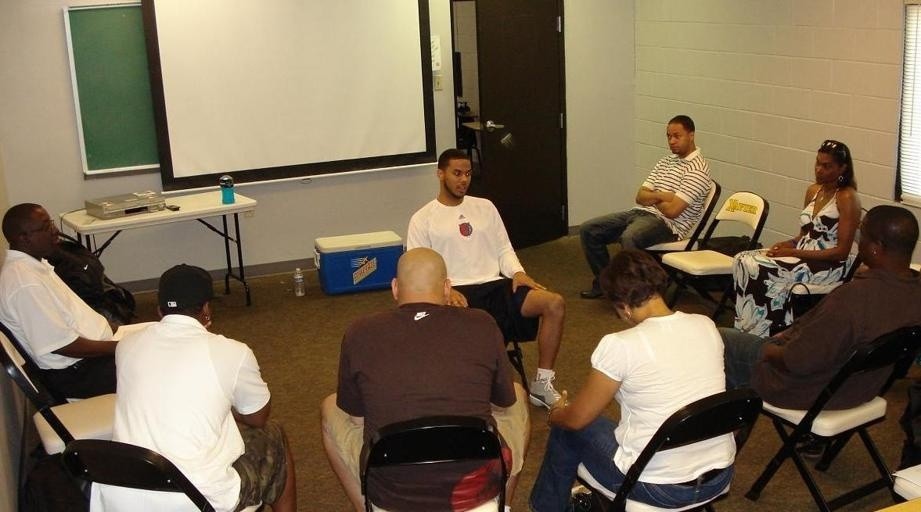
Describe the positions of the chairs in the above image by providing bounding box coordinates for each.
[1,324,116,511]
[576,387,763,512]
[506,338,530,397]
[892,464,921,500]
[642,179,722,286]
[362,415,506,512]
[747,326,921,512]
[662,191,769,318]
[61,440,264,512]
[711,208,870,335]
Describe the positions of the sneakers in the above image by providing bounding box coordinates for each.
[529,379,572,410]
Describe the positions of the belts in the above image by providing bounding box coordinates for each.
[676,467,725,486]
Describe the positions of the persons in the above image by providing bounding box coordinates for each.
[528,249,737,510]
[322,247,531,512]
[114,264,296,512]
[733,138,861,341]
[580,116,711,298]
[0,203,119,395]
[716,205,921,408]
[407,148,565,409]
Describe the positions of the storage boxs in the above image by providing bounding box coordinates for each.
[315,230,404,295]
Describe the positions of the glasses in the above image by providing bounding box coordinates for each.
[820,140,848,158]
[23,219,55,235]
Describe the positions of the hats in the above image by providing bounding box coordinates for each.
[158,262,214,309]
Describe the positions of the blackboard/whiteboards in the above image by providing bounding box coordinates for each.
[63,1,161,179]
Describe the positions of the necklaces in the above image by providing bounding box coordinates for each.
[820,186,837,201]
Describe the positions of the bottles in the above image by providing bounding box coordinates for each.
[219,175,235,204]
[294,268,305,296]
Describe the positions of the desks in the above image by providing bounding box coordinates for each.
[462,123,481,169]
[58,192,257,306]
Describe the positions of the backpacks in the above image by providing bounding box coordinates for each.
[44,233,136,325]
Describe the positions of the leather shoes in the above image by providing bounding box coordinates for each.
[581,285,606,300]
